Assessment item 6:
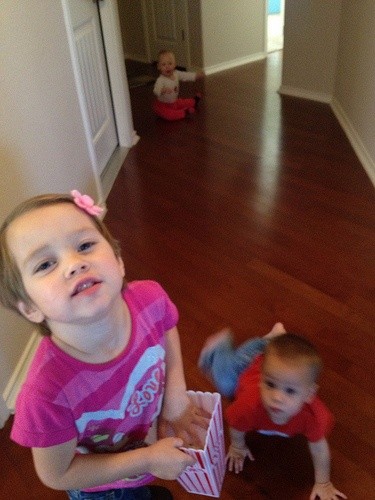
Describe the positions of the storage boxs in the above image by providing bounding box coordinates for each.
[142,391,228,498]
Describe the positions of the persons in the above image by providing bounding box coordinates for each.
[0,190,209,500]
[152,48,206,120]
[199,322,347,500]
